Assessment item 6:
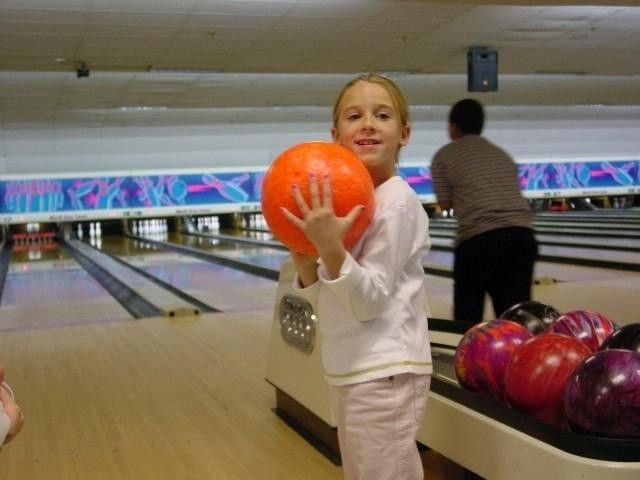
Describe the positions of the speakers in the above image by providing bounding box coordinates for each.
[466,49,498,93]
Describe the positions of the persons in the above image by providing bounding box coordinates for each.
[276,72,438,480]
[0,366,26,451]
[428,95,541,326]
